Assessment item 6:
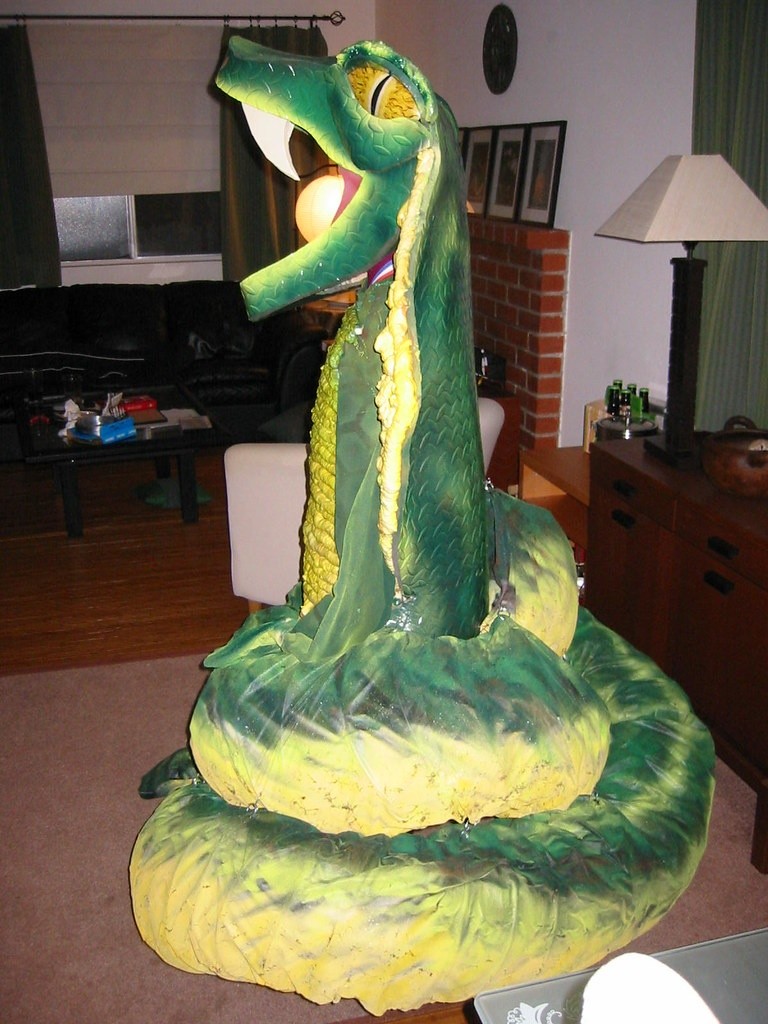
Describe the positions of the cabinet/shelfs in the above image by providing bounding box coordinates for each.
[586,432,768,843]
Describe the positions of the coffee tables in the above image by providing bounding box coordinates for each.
[20,384,234,539]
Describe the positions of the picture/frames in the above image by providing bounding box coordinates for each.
[458,119,567,228]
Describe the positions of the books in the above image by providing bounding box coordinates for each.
[94,394,157,412]
[180,415,212,431]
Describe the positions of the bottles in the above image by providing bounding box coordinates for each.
[603,379,655,424]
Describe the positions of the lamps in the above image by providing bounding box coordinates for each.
[593,152,768,467]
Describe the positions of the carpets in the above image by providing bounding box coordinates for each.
[0,649,768,1024]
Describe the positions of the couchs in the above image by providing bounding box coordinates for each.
[1,281,331,470]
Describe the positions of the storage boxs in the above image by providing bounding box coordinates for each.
[583,399,614,456]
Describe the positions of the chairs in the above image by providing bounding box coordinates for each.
[223,389,517,617]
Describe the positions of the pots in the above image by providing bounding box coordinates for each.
[701,415,768,501]
